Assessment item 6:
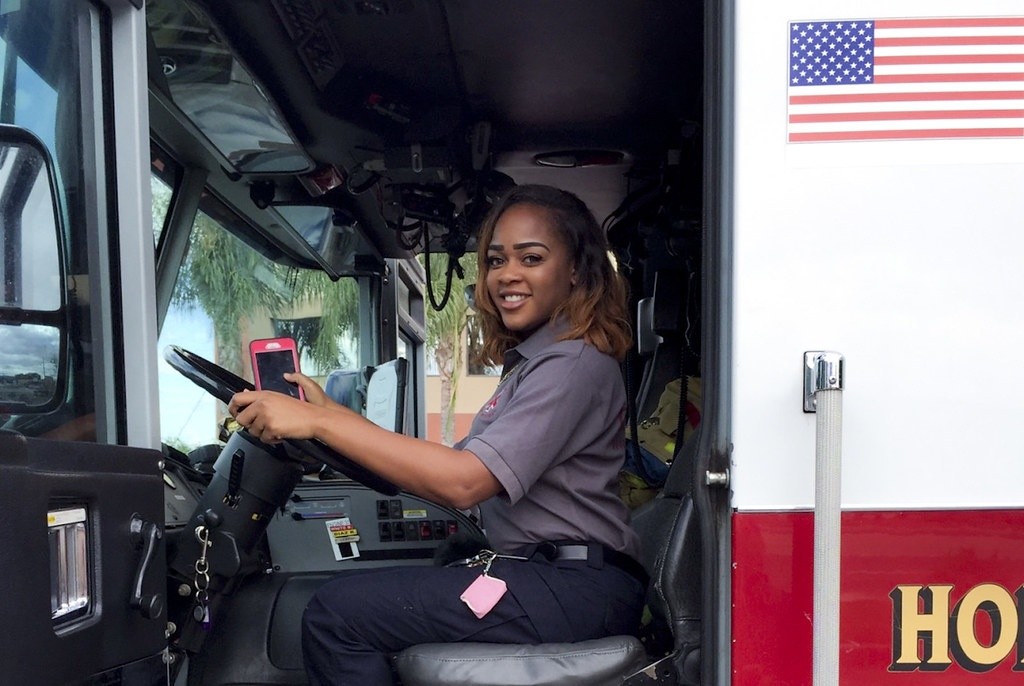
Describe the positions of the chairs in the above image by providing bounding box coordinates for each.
[390,427,700,686]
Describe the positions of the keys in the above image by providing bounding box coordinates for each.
[445,547,497,568]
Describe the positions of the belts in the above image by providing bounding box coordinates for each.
[536,542,651,595]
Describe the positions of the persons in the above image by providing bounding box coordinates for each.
[228,185,649,686]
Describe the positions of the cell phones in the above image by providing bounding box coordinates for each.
[248,338,306,403]
